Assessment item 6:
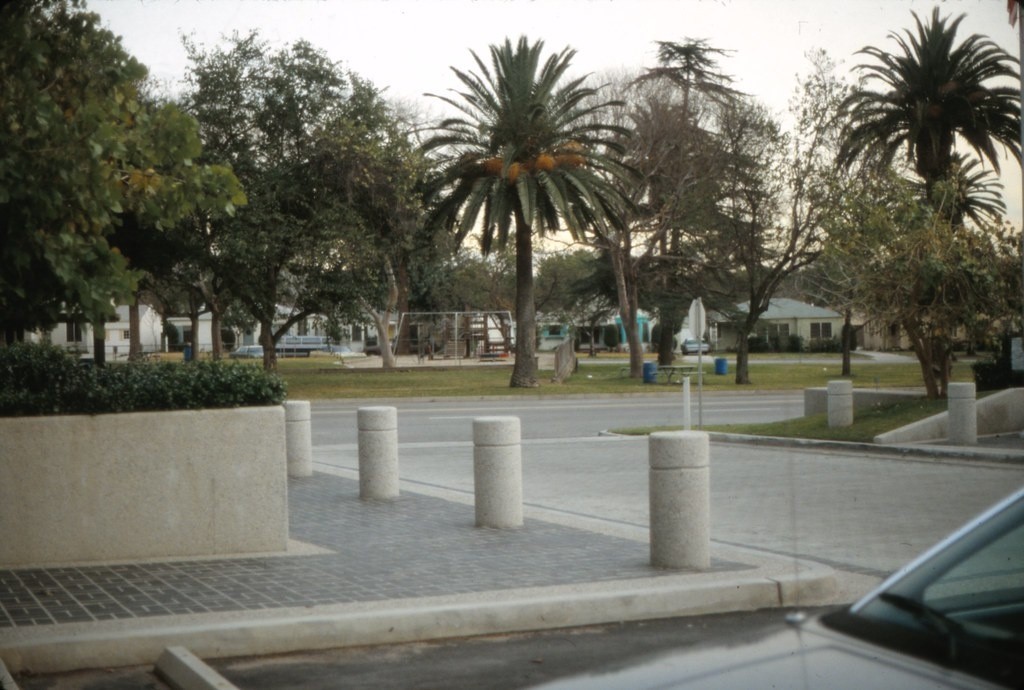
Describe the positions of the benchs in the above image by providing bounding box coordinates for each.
[650,365,707,386]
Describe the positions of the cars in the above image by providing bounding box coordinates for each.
[230,344,279,359]
[680,337,711,355]
[323,344,356,356]
[517,490,1023,690]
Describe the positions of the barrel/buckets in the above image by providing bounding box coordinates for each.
[715,359,727,374]
[643,363,657,382]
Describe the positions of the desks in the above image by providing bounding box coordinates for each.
[659,366,694,386]
